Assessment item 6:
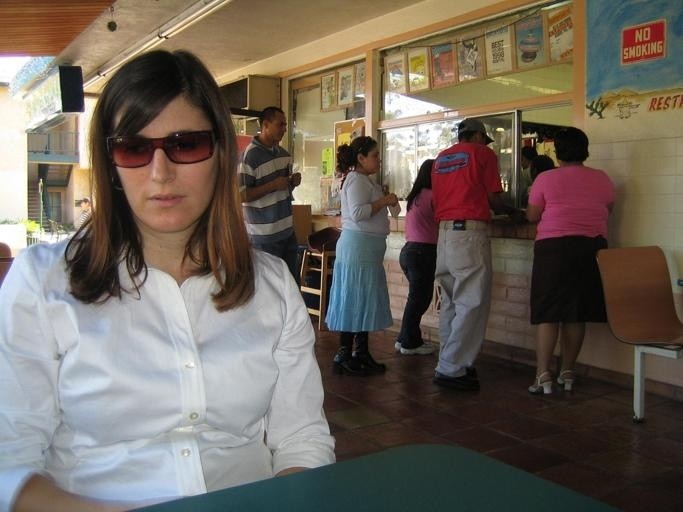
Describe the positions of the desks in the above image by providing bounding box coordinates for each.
[124,443,619,512]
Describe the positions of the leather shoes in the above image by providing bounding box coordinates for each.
[433,367,479,392]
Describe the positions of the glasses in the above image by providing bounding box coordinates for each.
[106,129,219,168]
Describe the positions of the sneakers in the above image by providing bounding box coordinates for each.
[395,340,435,354]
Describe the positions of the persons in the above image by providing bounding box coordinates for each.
[430,117,527,391]
[236,106,302,293]
[521,155,556,209]
[324,136,399,376]
[77,198,93,231]
[524,126,617,395]
[395,159,440,356]
[1,49,336,512]
[500,147,537,207]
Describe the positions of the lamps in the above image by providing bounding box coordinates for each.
[82,0,233,89]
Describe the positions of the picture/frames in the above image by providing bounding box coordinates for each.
[321,1,574,113]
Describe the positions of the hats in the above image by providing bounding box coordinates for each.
[458,117,494,144]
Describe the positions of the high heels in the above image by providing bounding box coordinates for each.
[351,351,386,372]
[528,370,552,394]
[333,356,368,376]
[556,368,575,391]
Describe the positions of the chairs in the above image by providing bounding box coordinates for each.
[596,245,682,424]
[300,227,342,331]
